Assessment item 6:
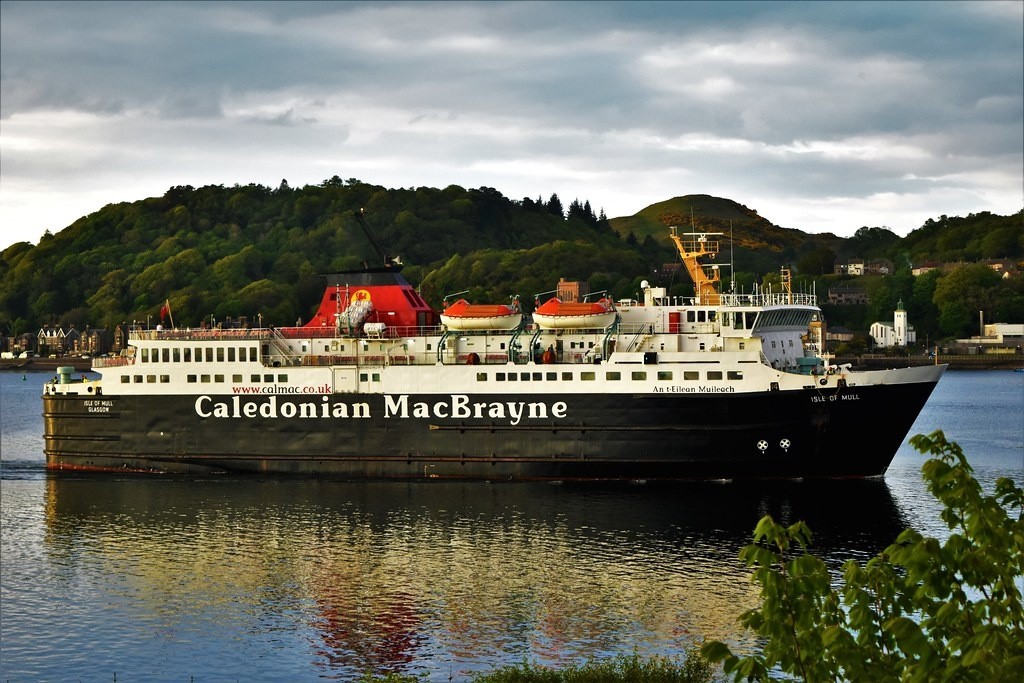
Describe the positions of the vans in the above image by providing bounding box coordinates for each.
[1,352,17,359]
[19,351,35,358]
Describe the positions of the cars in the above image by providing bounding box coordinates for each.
[34,354,108,359]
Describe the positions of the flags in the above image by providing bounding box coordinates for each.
[160,303,169,321]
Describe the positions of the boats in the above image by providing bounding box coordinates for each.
[531,298,618,330]
[41,229,950,482]
[438,298,523,330]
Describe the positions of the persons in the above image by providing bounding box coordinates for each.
[156,323,163,339]
[826,367,836,377]
[54,376,58,384]
[81,375,89,383]
[809,366,817,375]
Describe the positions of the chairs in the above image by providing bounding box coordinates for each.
[320,356,414,365]
[485,355,508,363]
[104,359,127,367]
[157,331,270,339]
[456,355,468,363]
